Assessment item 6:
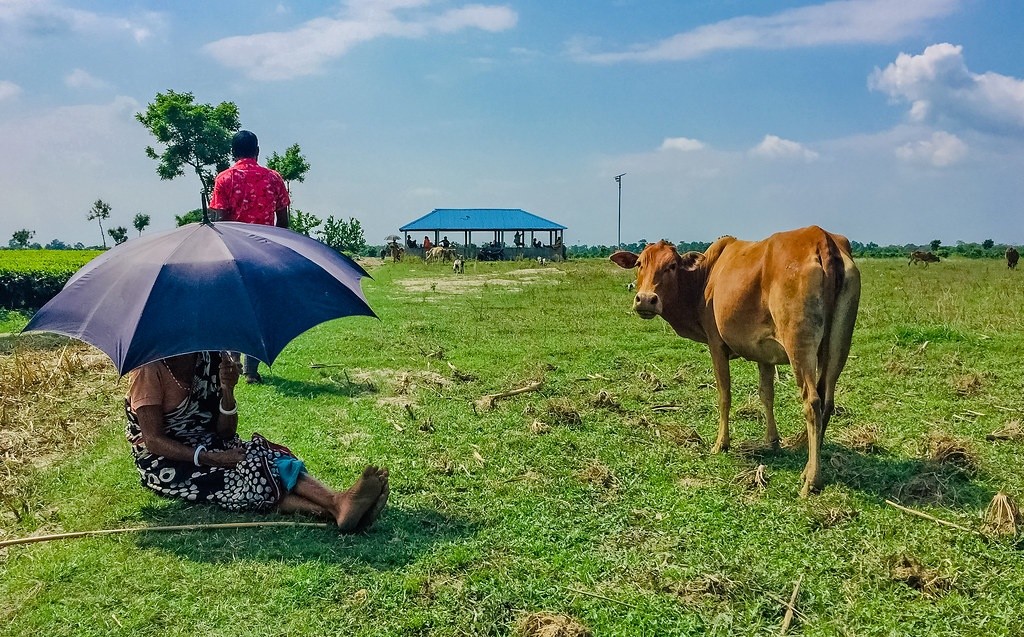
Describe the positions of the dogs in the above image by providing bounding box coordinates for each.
[453,259,465,274]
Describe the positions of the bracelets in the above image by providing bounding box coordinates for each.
[194,445,207,467]
[219,397,237,415]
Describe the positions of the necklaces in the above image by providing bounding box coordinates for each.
[163,359,190,391]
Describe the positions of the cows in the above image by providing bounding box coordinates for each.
[609,225,861,499]
[426,246,446,262]
[908,251,941,269]
[1005,247,1019,269]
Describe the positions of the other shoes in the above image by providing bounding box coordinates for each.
[246,372,261,383]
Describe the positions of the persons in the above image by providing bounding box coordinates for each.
[391,232,562,252]
[208,130,291,383]
[124,350,390,536]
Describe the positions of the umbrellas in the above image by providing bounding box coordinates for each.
[18,194,383,374]
[384,235,401,241]
